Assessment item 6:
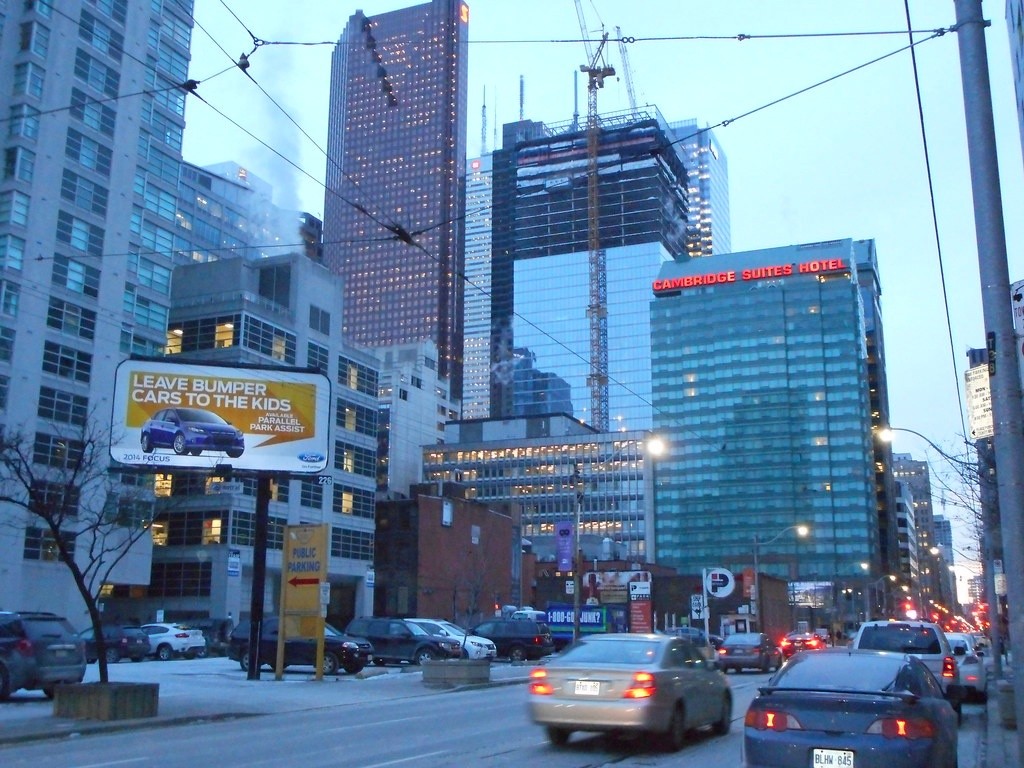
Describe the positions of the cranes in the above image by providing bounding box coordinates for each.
[573,1,615,433]
[616,26,641,121]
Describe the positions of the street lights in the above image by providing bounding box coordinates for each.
[570,427,911,638]
[880,428,1003,676]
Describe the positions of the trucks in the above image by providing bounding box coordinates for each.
[509,604,627,651]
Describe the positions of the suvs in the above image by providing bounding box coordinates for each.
[856,620,961,721]
[181,619,235,658]
[0,611,86,700]
[228,614,374,674]
[345,615,461,666]
[468,619,555,662]
[77,619,205,664]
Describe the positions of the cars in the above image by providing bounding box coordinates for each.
[719,628,831,673]
[141,408,246,458]
[943,632,986,704]
[394,618,497,663]
[745,646,960,767]
[667,628,725,649]
[526,630,734,749]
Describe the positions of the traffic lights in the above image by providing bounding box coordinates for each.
[903,603,911,611]
